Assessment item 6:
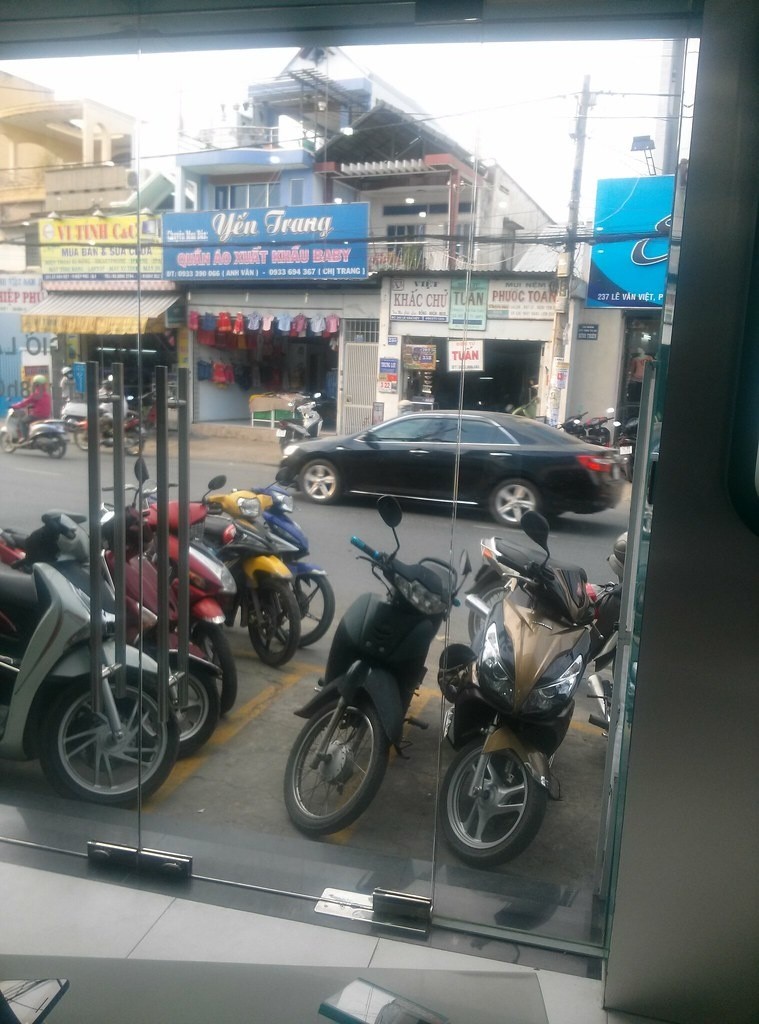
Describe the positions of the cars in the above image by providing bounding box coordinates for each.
[277,409,624,528]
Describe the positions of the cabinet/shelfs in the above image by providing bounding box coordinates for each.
[250,395,308,429]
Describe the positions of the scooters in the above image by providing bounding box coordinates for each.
[276,390,324,453]
[60,373,129,434]
[0,403,70,460]
[554,407,640,482]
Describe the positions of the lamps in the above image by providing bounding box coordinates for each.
[92,211,106,219]
[632,135,658,177]
[48,212,58,220]
[140,205,155,218]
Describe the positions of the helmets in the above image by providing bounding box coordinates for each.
[33,375,47,385]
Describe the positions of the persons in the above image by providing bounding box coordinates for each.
[627,345,655,438]
[60,366,75,400]
[9,375,52,443]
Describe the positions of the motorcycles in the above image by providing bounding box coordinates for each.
[464,532,627,686]
[1,453,335,810]
[436,503,627,870]
[283,491,462,836]
[72,395,148,457]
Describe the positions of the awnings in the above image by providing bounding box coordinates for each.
[20,294,180,334]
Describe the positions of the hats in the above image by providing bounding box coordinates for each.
[61,367,72,375]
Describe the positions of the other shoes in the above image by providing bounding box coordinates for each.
[18,437,28,443]
